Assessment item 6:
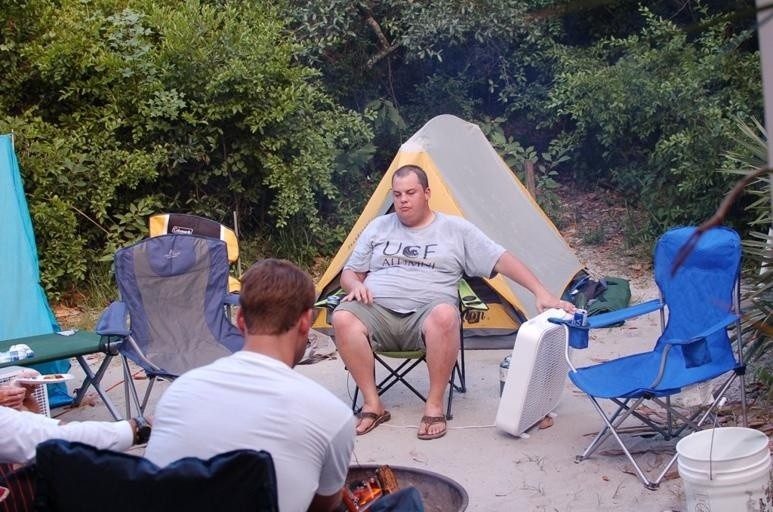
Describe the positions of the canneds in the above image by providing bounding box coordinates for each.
[328,296,340,304]
[572,309,587,326]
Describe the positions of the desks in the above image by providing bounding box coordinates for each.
[0,330,125,424]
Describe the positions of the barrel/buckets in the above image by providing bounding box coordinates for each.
[676,398,773,512]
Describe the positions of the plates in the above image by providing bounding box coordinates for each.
[17,373,73,384]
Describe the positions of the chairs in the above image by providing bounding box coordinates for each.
[546,224,747,491]
[95,212,243,424]
[314,278,489,421]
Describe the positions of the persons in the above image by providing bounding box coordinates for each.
[331,165,577,440]
[143,258,356,512]
[0,406,154,465]
[0,371,40,414]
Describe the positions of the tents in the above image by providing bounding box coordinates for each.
[310,114,596,350]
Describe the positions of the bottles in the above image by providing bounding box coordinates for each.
[0,350,34,364]
[499,355,512,398]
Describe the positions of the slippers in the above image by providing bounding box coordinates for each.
[355,411,391,435]
[418,414,447,439]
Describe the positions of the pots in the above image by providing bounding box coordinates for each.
[345,464,468,511]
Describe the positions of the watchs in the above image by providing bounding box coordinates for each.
[131,416,152,445]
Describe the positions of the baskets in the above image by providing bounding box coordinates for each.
[0,366,50,419]
